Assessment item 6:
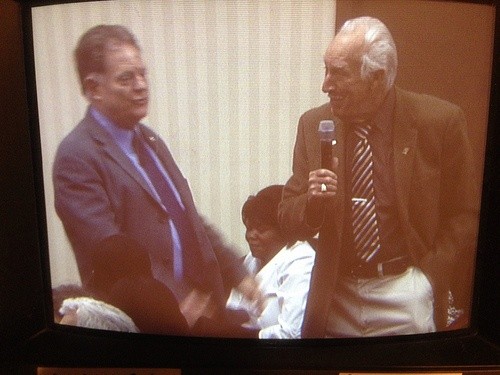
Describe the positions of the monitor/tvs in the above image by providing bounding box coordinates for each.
[21,0,500,375]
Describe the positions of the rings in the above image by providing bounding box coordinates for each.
[321,184,327,192]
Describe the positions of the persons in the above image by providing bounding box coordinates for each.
[53,285,91,324]
[59,297,140,333]
[226,185,315,339]
[278,16,480,337]
[52,26,265,328]
[86,233,193,337]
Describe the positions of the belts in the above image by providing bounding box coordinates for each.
[338,255,412,281]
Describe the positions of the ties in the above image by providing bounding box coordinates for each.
[350,122,380,262]
[130,130,196,288]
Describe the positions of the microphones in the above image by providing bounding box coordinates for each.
[319,120,336,170]
